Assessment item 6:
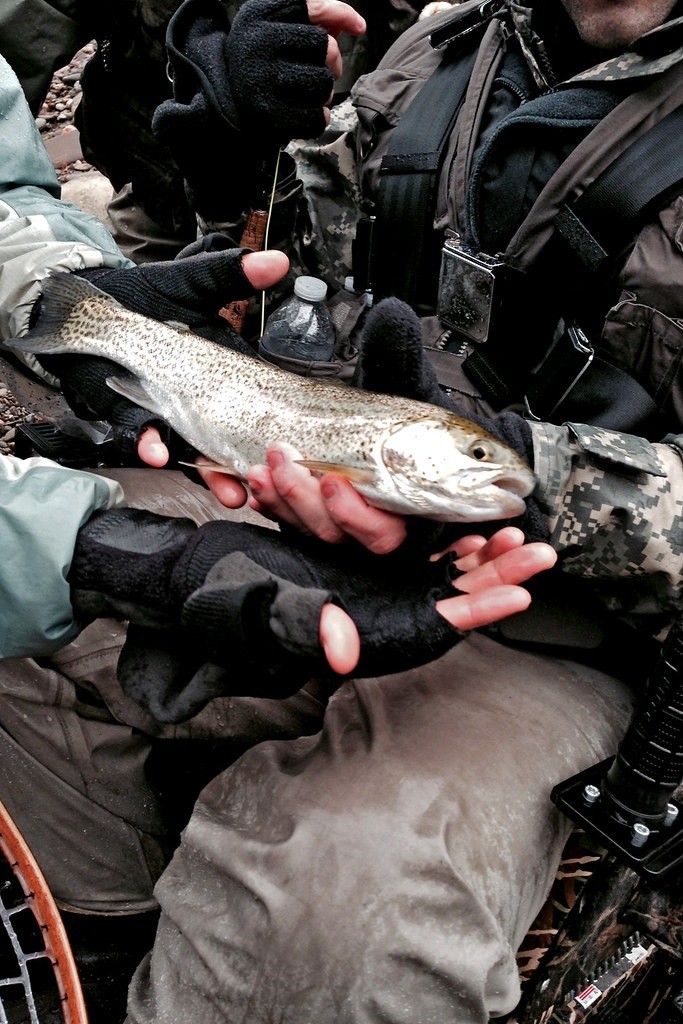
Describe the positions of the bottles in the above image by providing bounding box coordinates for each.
[261,275,334,361]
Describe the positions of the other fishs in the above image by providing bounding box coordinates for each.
[4,264,536,526]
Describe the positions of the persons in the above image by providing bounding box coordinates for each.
[1,1,683,1024]
